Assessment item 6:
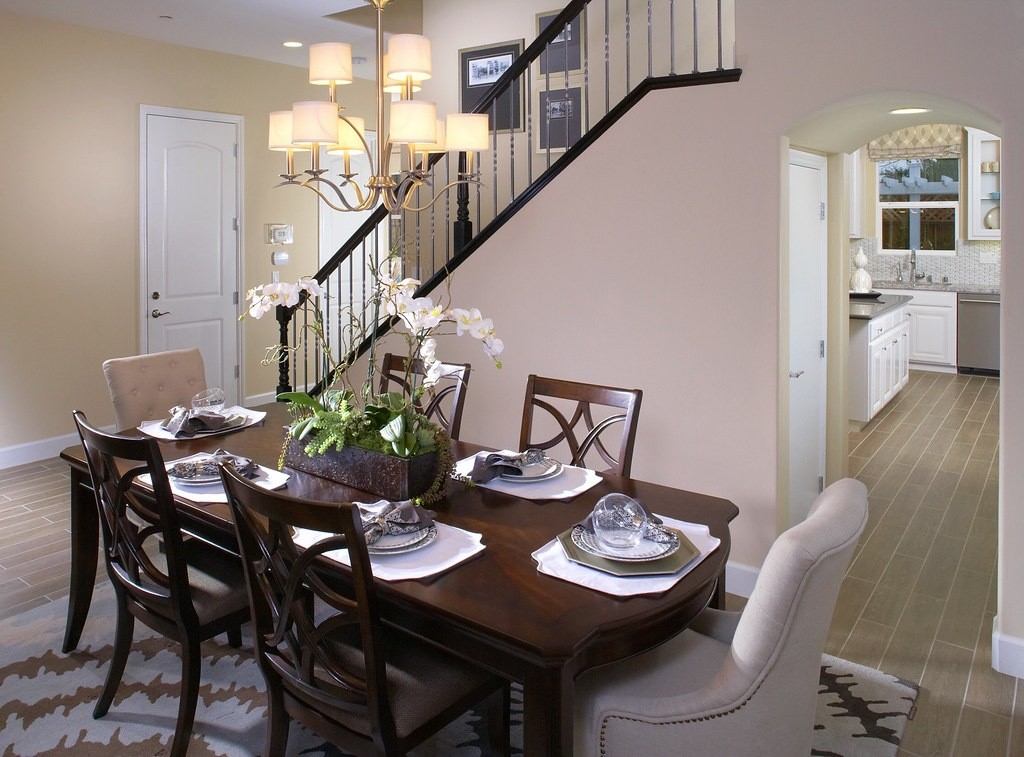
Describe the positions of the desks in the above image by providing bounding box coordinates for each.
[59,401,740,757]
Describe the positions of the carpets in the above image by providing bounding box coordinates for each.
[0,573,920,757]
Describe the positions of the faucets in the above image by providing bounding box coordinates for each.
[909,248,924,282]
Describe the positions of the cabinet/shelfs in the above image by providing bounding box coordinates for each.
[871,287,958,374]
[963,126,1001,241]
[848,302,910,423]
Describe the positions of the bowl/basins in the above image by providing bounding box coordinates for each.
[991,192,1000,199]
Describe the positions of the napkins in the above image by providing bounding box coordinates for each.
[467,448,548,485]
[166,406,228,439]
[166,448,261,482]
[351,500,438,545]
[573,497,678,544]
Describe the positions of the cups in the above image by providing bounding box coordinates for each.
[592,493,647,552]
[191,388,226,412]
[981,162,1000,172]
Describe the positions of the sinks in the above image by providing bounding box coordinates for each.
[879,281,953,288]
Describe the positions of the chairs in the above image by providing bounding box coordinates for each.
[71,348,870,757]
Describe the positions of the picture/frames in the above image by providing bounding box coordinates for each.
[536,82,585,153]
[535,6,587,80]
[458,38,525,135]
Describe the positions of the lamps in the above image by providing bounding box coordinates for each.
[268,0,489,213]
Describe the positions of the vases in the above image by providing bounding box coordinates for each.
[282,425,446,502]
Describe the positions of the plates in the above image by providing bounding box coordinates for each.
[984,206,1000,229]
[159,410,249,433]
[570,522,681,562]
[495,457,564,483]
[332,519,439,555]
[167,455,248,487]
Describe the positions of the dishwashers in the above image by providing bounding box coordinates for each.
[957,293,1000,375]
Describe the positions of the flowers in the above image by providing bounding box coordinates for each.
[237,234,504,506]
